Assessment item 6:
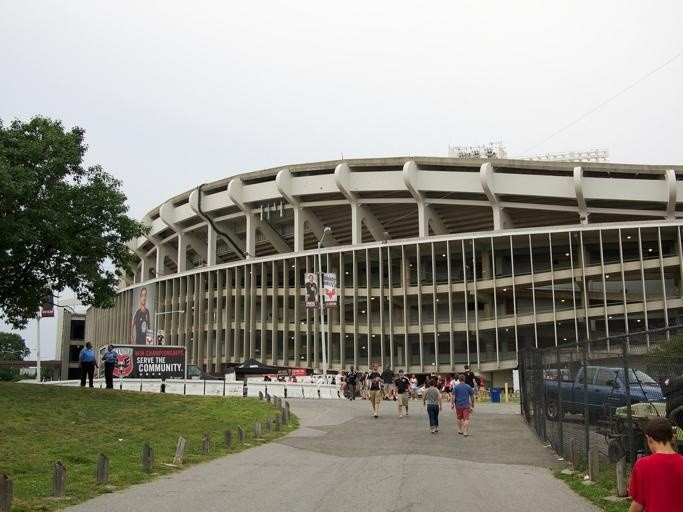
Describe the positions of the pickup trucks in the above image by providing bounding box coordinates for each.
[531,364,666,422]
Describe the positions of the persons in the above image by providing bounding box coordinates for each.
[626,417,683,512]
[101,344,118,389]
[156,331,164,345]
[305,275,317,301]
[77,341,98,387]
[263,375,271,381]
[279,376,285,382]
[131,287,150,344]
[312,362,479,436]
[290,375,296,382]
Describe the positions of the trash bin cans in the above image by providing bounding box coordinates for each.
[490,388,500,402]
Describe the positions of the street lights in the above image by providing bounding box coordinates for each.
[317,226,333,383]
[153,310,184,345]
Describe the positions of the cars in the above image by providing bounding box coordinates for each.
[613,397,682,447]
